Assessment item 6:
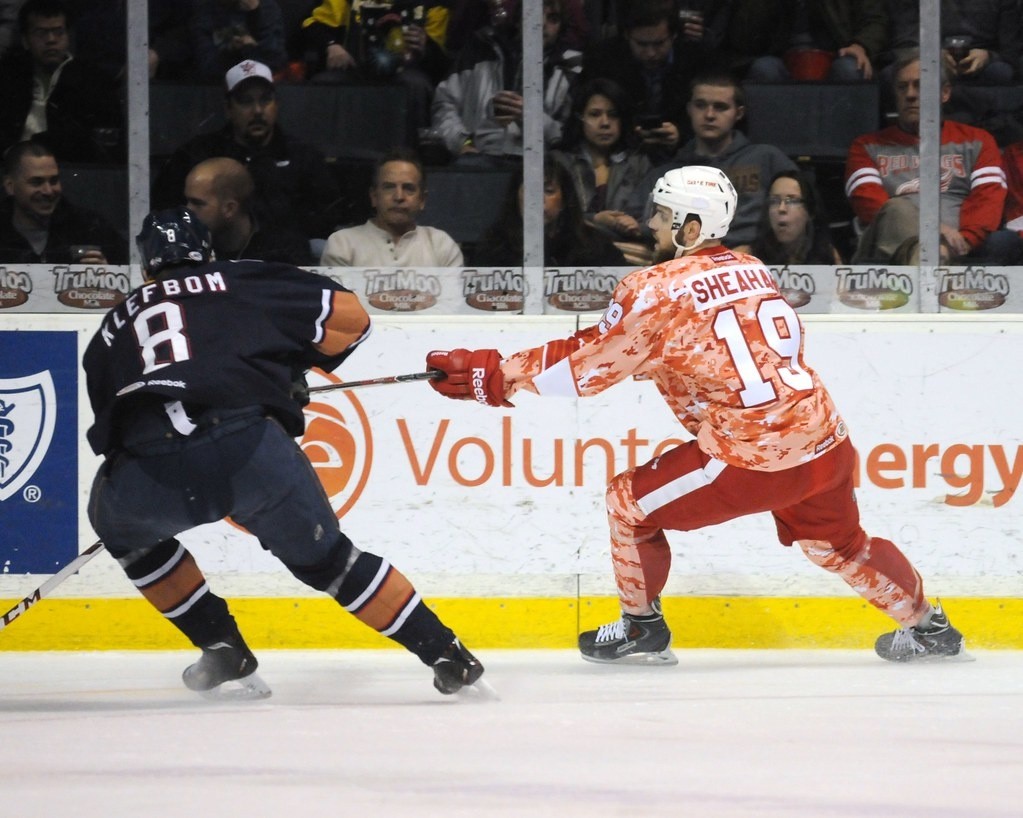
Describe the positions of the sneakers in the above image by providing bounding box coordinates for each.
[182,625,271,700]
[875,596,976,665]
[431,638,490,697]
[578,598,678,665]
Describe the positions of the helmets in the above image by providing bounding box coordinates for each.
[135,208,213,275]
[653,165,738,251]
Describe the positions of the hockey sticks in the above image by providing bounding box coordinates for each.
[302,363,450,394]
[0,536,107,634]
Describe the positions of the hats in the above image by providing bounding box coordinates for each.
[225,59,273,91]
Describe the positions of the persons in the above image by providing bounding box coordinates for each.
[426,165,968,661]
[81,206,486,692]
[0,0,1023,267]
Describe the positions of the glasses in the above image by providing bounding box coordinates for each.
[769,196,803,208]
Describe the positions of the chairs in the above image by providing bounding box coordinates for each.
[417,167,522,243]
[124,83,436,165]
[739,82,886,165]
[56,164,129,261]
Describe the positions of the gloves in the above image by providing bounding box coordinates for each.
[425,348,513,409]
